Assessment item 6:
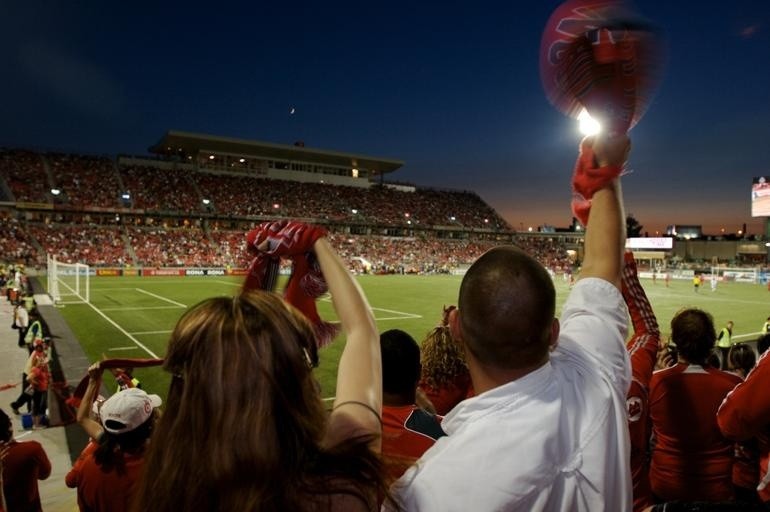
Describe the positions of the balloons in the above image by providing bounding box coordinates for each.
[540,0,664,135]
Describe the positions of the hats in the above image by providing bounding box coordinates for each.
[33,337,50,347]
[100,387,162,435]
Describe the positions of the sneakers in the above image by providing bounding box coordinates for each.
[10,402,20,414]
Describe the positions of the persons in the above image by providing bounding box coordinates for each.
[2,120,770,288]
[0,252,767,512]
[382,120,634,512]
[138,218,380,512]
